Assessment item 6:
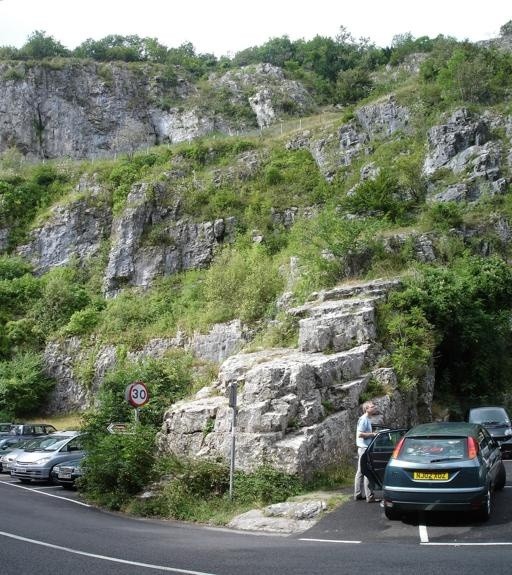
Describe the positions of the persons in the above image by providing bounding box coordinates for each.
[353,401,381,502]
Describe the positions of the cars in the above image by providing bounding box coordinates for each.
[50,452,87,491]
[467,406,512,460]
[358,423,506,520]
[0,422,57,470]
[11,431,88,485]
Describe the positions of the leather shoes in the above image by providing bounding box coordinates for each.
[355,497,365,501]
[367,498,381,503]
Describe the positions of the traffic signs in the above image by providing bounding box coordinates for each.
[125,381,150,409]
[106,421,139,435]
